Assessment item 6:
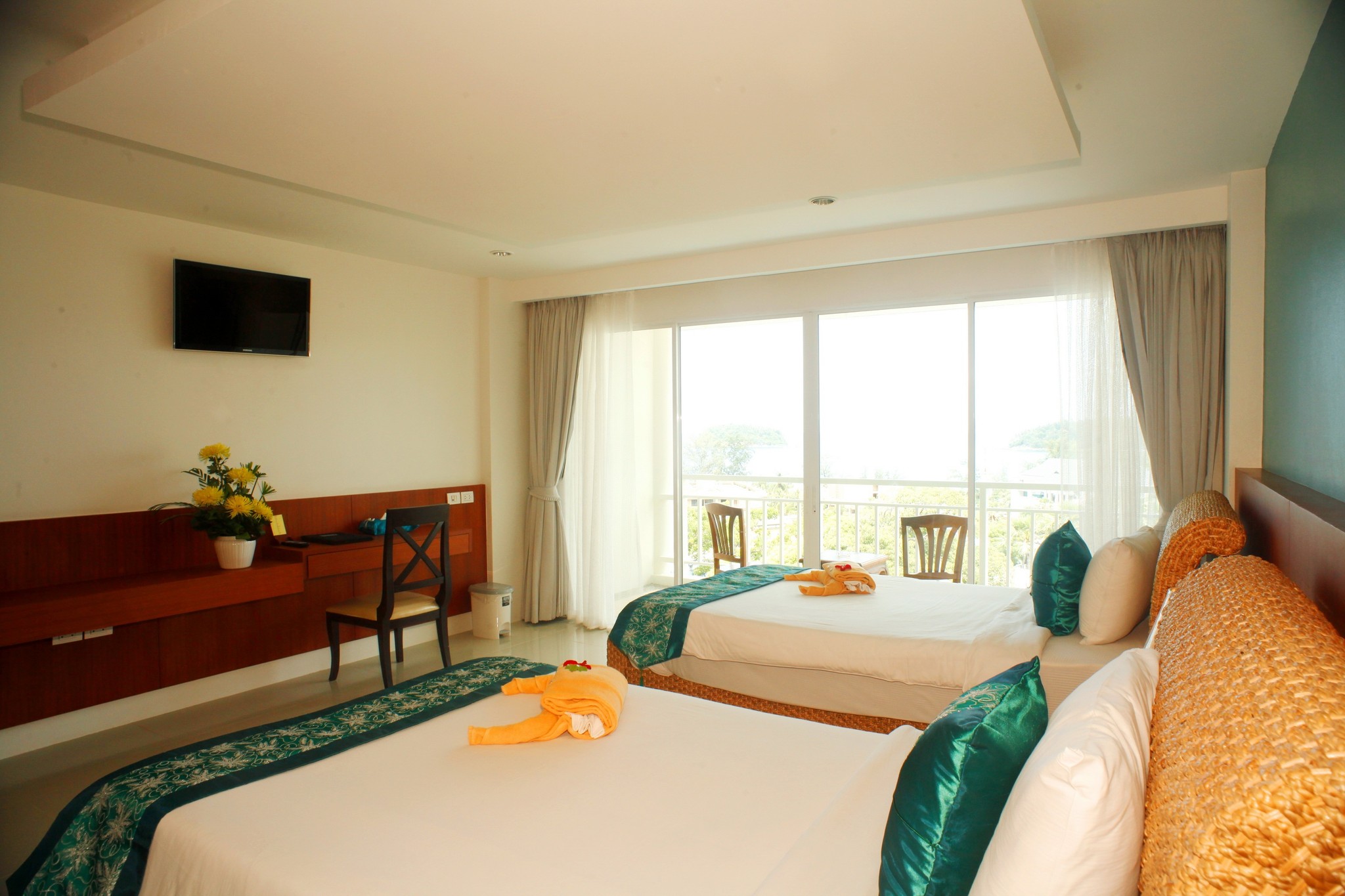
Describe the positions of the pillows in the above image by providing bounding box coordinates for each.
[878,655,1049,896]
[968,649,1161,896]
[1032,520,1092,636]
[1078,526,1161,646]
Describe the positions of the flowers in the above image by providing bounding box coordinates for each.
[148,442,277,537]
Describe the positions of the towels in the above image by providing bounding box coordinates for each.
[468,662,631,749]
[782,559,878,598]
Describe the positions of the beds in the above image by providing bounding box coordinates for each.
[606,491,1246,735]
[5,553,1345,896]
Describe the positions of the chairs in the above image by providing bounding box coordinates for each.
[326,503,452,690]
[705,502,747,575]
[901,514,967,583]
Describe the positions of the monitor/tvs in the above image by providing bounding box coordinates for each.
[172,259,310,357]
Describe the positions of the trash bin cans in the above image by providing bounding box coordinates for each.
[469,582,514,639]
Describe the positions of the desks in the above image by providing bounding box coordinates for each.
[0,558,307,644]
[273,527,470,582]
[799,549,889,575]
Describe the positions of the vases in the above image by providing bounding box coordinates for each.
[214,536,256,569]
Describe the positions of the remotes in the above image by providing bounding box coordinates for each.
[281,540,308,548]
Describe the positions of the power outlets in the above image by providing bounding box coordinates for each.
[460,491,474,504]
